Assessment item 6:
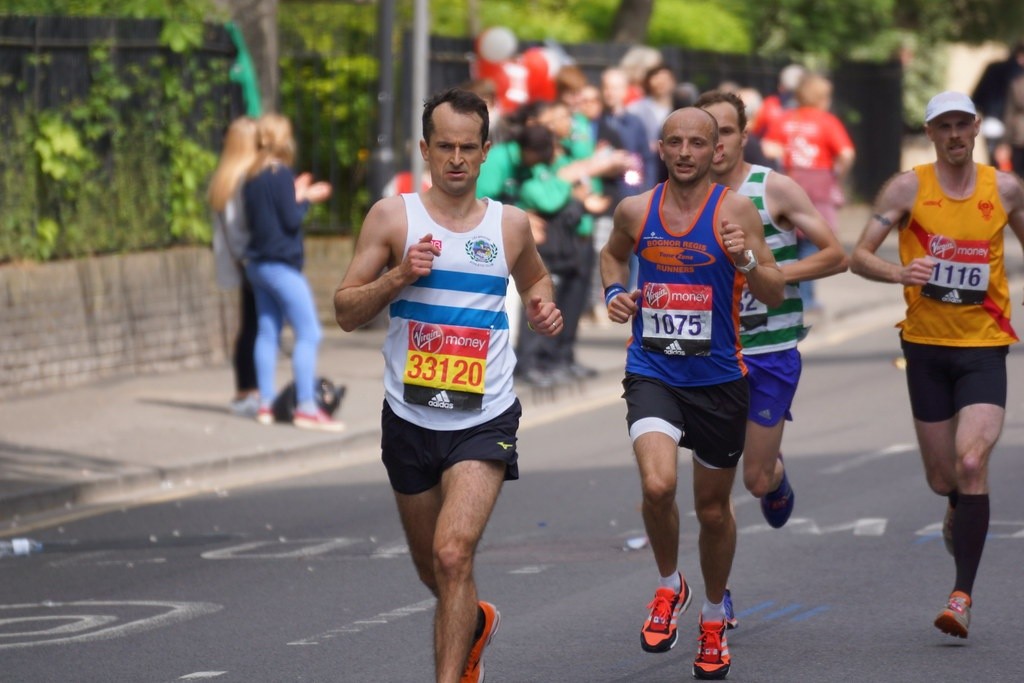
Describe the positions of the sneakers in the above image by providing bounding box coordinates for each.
[723,589,738,627]
[933,600,971,638]
[693,606,731,679]
[640,568,694,652]
[458,603,502,683]
[943,496,954,555]
[760,454,794,528]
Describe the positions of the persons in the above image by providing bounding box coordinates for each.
[599,106,785,679]
[333,87,564,683]
[475,66,857,391]
[694,90,850,630]
[970,38,1024,181]
[203,112,344,433]
[851,92,1024,639]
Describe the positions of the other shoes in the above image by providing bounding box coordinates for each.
[294,408,345,432]
[549,364,576,384]
[570,360,598,379]
[234,395,260,417]
[258,410,273,425]
[524,361,552,389]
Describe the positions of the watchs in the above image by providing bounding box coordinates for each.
[733,249,758,274]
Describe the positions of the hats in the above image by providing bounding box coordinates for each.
[925,92,977,124]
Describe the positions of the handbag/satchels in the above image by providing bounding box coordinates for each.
[275,374,345,422]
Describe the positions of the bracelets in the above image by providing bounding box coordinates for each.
[527,321,536,333]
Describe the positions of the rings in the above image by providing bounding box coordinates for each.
[551,322,558,329]
